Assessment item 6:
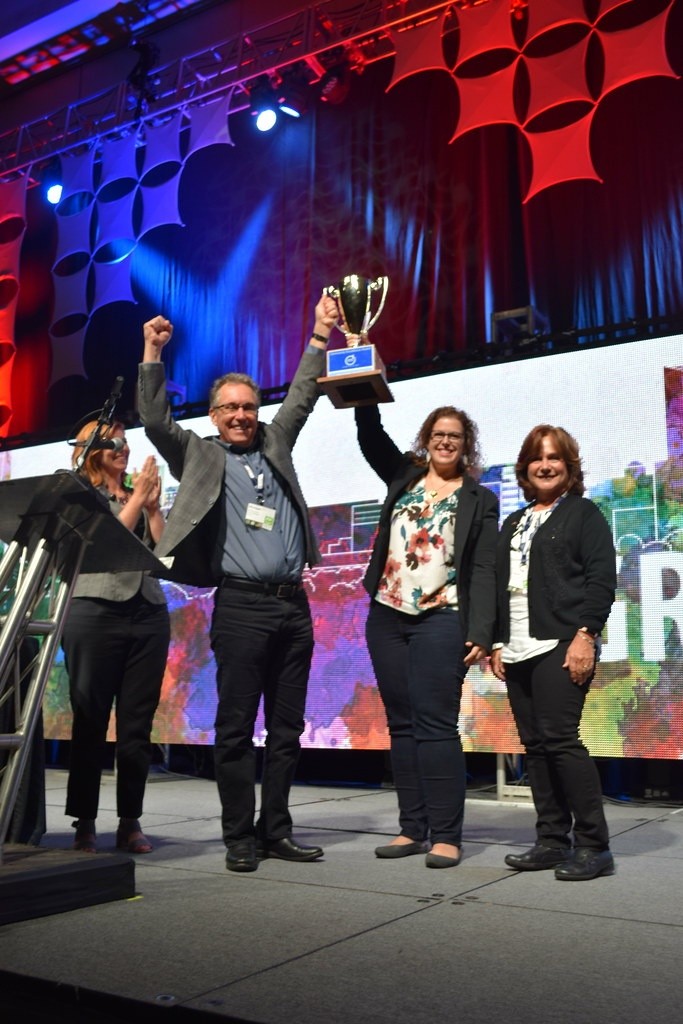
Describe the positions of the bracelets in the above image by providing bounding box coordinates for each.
[311,332,329,343]
[576,632,594,645]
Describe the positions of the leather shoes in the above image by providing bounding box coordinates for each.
[505,845,571,870]
[225,843,257,871]
[256,836,324,861]
[555,847,615,879]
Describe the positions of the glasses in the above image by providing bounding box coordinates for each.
[430,430,464,443]
[213,401,259,414]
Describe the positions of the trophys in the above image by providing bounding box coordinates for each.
[317,274,395,409]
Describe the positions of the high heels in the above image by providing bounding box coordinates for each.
[116,820,153,853]
[71,821,97,854]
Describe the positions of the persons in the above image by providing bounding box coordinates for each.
[58,418,170,854]
[345,330,500,863]
[134,295,338,869]
[490,424,618,881]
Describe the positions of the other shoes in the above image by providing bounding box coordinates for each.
[375,838,432,858]
[425,853,459,868]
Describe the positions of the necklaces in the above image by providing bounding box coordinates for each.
[429,470,458,497]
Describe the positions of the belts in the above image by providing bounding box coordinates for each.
[224,578,303,598]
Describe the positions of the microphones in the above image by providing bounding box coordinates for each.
[69,437,124,451]
